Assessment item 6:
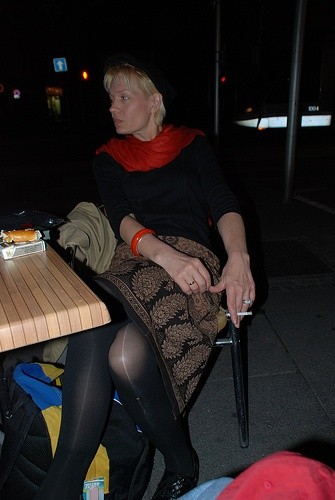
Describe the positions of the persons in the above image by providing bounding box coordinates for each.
[35,51,257,500]
[172,450,335,500]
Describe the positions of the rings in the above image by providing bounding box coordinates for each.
[242,299,253,304]
[188,280,196,285]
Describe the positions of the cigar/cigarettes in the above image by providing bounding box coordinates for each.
[225,311,253,317]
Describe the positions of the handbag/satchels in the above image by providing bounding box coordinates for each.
[1,362,153,500]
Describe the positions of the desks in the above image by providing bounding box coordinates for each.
[0,223,111,353]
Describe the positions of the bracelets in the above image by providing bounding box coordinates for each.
[130,228,157,257]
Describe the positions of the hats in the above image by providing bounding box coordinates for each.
[118,57,171,109]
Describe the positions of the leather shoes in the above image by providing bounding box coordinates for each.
[151,450,199,500]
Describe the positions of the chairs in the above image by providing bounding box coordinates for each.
[214,303,252,448]
